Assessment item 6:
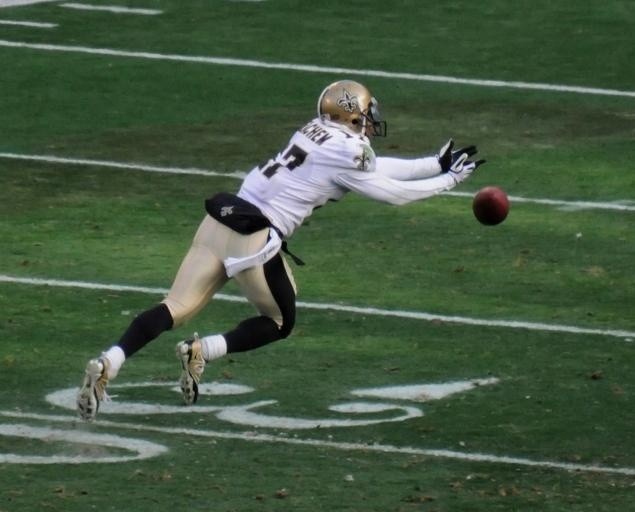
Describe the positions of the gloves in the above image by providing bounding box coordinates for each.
[435,137,487,185]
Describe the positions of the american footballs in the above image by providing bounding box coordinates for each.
[472,187,509,225]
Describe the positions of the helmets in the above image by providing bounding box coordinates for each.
[317,80,387,138]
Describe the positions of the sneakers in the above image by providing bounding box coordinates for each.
[77,351,111,422]
[176,331,208,405]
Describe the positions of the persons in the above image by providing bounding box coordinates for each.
[76,79,487,422]
